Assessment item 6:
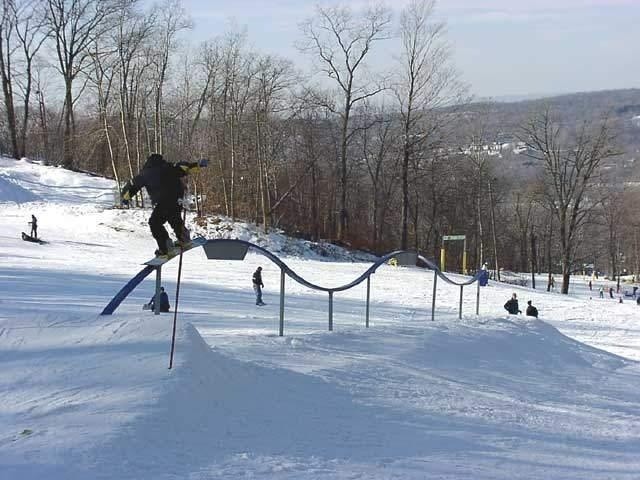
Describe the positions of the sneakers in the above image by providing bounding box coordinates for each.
[174,240,184,245]
[155,249,167,255]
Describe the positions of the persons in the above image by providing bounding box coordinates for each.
[479,261,640,319]
[28,215,37,239]
[120,154,208,260]
[252,267,266,306]
[148,287,170,312]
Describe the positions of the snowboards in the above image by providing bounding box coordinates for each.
[144,238,207,266]
